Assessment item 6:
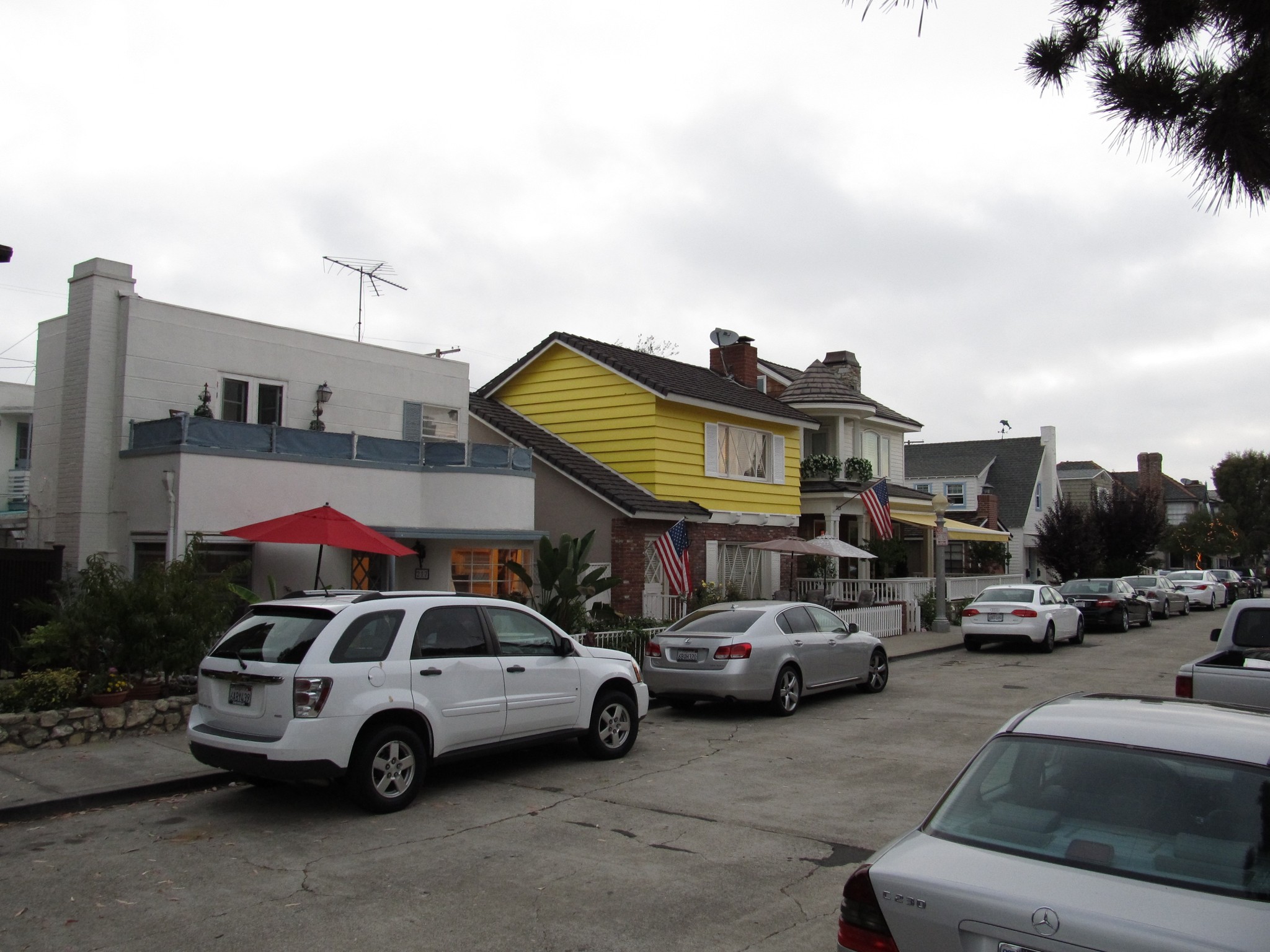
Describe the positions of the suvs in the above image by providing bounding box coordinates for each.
[184,587,651,817]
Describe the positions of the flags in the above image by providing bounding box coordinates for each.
[654,519,695,603]
[860,476,895,541]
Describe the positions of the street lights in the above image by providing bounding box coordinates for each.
[931,490,953,634]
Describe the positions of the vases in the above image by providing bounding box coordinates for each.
[850,471,862,479]
[817,470,832,478]
[91,688,129,705]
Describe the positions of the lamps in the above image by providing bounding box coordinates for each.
[309,380,333,431]
[194,382,215,419]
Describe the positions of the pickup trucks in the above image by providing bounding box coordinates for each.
[1174,596,1270,714]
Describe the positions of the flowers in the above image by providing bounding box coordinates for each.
[845,457,873,483]
[801,453,842,485]
[89,668,133,694]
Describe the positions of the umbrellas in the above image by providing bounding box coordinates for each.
[221,502,418,591]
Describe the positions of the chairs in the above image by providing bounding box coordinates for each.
[1099,588,1107,593]
[857,589,876,609]
[774,590,796,601]
[821,594,836,611]
[807,590,829,606]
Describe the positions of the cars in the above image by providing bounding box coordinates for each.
[1119,574,1190,620]
[833,690,1269,952]
[1058,576,1153,633]
[1165,569,1229,611]
[960,584,1086,655]
[1203,569,1251,605]
[1226,569,1264,599]
[640,598,891,718]
